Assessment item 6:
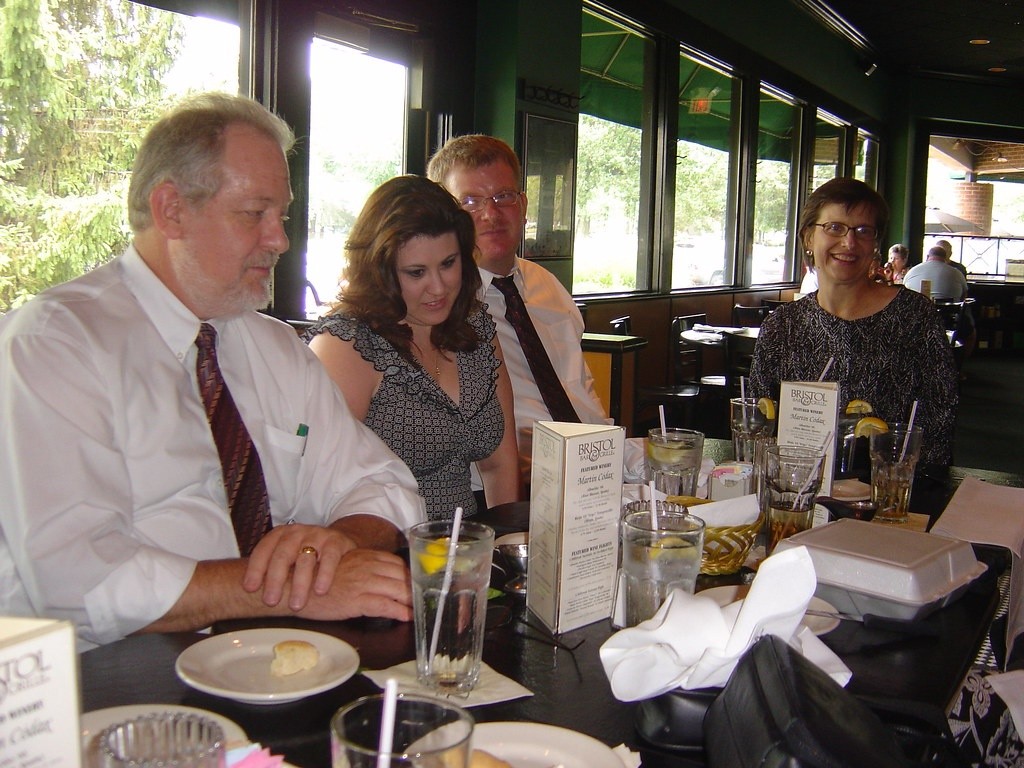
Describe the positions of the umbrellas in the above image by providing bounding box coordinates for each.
[926,207,984,233]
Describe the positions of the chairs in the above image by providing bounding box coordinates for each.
[733,301,770,328]
[722,329,757,437]
[931,299,968,331]
[671,313,745,437]
[609,316,700,438]
[760,299,788,312]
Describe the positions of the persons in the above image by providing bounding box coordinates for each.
[903,247,977,381]
[305,174,520,521]
[800,271,818,294]
[1,94,428,651]
[426,136,612,497]
[936,240,966,280]
[877,243,910,285]
[747,177,959,485]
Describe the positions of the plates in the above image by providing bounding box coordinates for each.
[401,720,629,768]
[694,583,841,637]
[176,626,362,706]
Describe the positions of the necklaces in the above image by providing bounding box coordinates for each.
[436,367,440,375]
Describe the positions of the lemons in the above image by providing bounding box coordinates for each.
[648,442,683,462]
[757,398,775,420]
[418,537,448,575]
[846,400,872,413]
[647,536,698,560]
[853,417,889,438]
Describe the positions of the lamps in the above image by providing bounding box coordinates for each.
[858,53,878,76]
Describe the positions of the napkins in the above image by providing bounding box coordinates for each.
[598,543,854,704]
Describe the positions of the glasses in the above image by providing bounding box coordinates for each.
[484,605,586,650]
[813,222,880,241]
[459,190,525,213]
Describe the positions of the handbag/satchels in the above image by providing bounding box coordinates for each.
[640,632,916,768]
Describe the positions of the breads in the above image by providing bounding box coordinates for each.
[271,640,319,677]
[469,749,514,768]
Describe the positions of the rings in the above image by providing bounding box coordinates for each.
[300,546,318,560]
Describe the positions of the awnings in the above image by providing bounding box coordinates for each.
[578,10,862,166]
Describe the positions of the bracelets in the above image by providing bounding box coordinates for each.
[887,280,891,282]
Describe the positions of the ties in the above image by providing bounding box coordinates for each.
[491,274,583,424]
[194,323,273,558]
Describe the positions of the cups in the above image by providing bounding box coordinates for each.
[331,692,476,767]
[620,501,707,629]
[408,519,496,694]
[88,714,229,767]
[648,428,705,498]
[730,397,827,557]
[869,423,924,524]
[834,406,862,476]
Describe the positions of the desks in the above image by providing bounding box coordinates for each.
[79,465,1024,768]
[679,327,762,440]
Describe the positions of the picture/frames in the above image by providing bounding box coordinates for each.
[518,110,574,260]
